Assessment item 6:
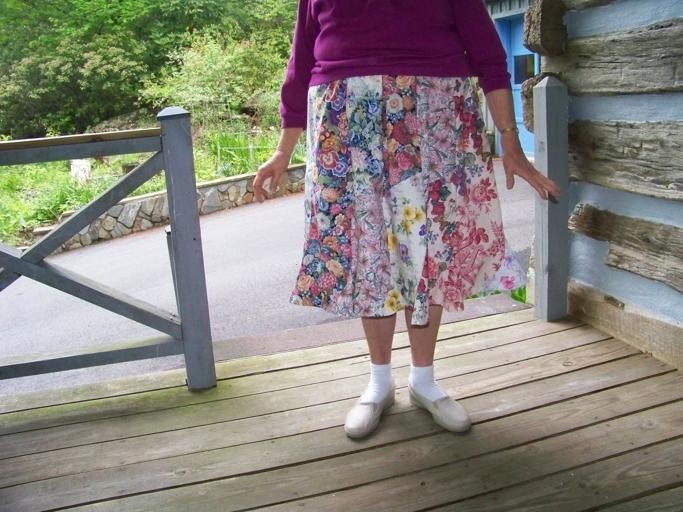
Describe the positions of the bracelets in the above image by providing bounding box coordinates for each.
[499,126,519,133]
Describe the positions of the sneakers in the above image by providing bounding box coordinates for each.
[409,385,471,433]
[345,376,394,438]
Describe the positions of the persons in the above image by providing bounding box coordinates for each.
[252,1,561,441]
